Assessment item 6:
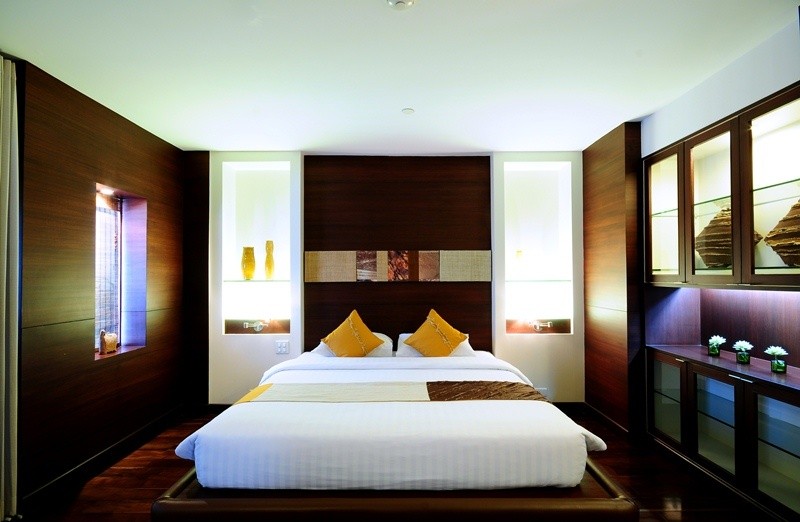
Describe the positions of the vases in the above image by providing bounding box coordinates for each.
[771,359,787,374]
[708,345,720,358]
[736,351,750,365]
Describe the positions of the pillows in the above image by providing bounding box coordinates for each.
[311,310,393,358]
[395,308,476,357]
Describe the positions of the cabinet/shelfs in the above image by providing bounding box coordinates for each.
[638,81,800,291]
[504,161,572,319]
[222,162,290,320]
[645,344,800,522]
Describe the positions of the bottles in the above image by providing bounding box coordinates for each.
[265,240,274,279]
[241,246,255,279]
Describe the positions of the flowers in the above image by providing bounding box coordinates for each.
[732,340,754,361]
[764,346,789,366]
[708,335,727,353]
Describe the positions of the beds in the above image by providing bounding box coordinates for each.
[174,319,606,490]
